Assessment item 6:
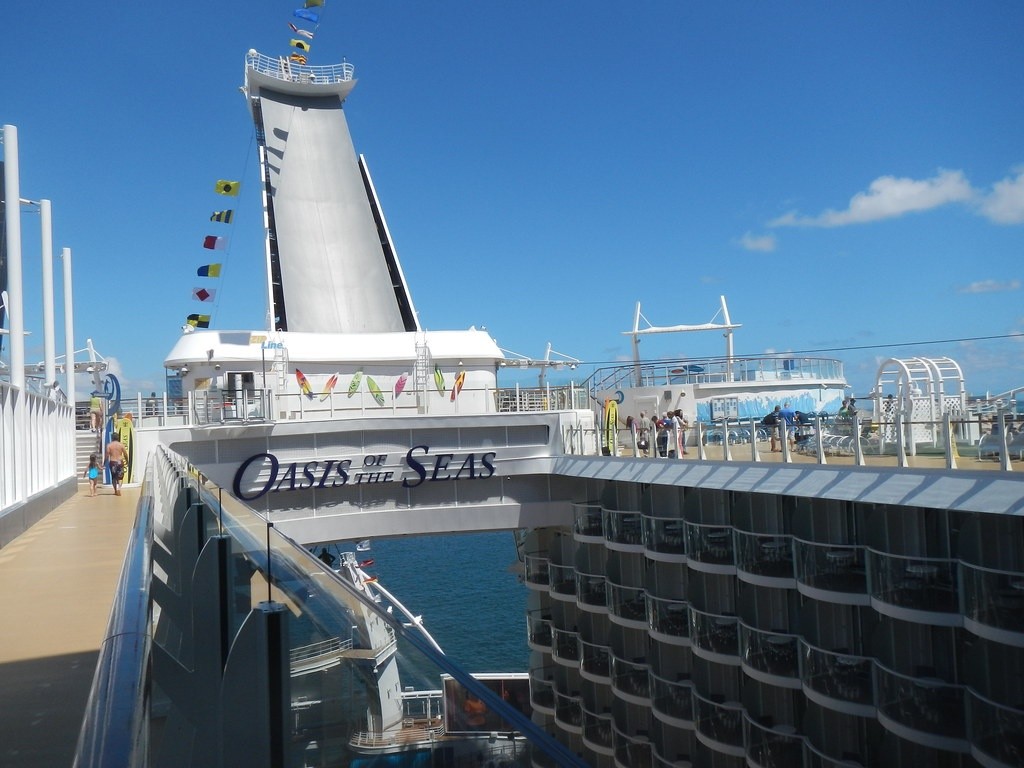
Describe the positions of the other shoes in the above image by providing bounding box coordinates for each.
[114,491,121,496]
[771,448,781,452]
[791,449,796,451]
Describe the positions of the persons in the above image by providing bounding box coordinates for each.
[794,411,815,440]
[769,406,781,451]
[90,391,102,433]
[778,401,797,452]
[104,432,129,496]
[884,395,893,415]
[626,409,689,457]
[318,547,336,568]
[848,399,858,416]
[146,391,158,416]
[839,401,848,417]
[83,455,103,496]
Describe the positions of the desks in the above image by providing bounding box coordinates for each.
[589,579,605,597]
[546,603,952,768]
[641,592,656,599]
[624,518,642,544]
[708,532,730,559]
[667,525,683,548]
[827,551,857,584]
[764,542,788,567]
[906,565,939,600]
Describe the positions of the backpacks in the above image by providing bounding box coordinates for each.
[761,415,775,425]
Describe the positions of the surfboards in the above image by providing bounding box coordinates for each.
[450,369,465,402]
[347,367,363,398]
[105,416,114,484]
[123,412,132,421]
[295,368,313,400]
[319,371,340,401]
[112,413,117,429]
[434,363,445,397]
[392,371,408,400]
[604,401,619,453]
[366,375,385,406]
[116,418,134,484]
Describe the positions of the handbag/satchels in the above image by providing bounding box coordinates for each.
[638,440,650,449]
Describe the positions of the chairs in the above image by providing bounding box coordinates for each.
[571,690,580,703]
[978,414,1024,463]
[756,536,785,569]
[705,417,768,446]
[603,707,611,713]
[592,508,602,535]
[622,513,641,543]
[538,560,549,579]
[901,558,925,600]
[635,728,648,736]
[708,693,729,727]
[546,675,554,687]
[831,544,850,579]
[676,753,690,761]
[707,527,727,559]
[796,414,875,457]
[664,519,683,549]
[758,715,777,753]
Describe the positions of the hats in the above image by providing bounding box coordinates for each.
[850,398,857,402]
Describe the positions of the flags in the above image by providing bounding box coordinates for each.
[186,314,211,328]
[192,287,216,303]
[197,263,222,277]
[290,40,310,50]
[216,180,240,197]
[288,0,324,40]
[290,52,306,64]
[211,209,233,223]
[202,235,227,251]
